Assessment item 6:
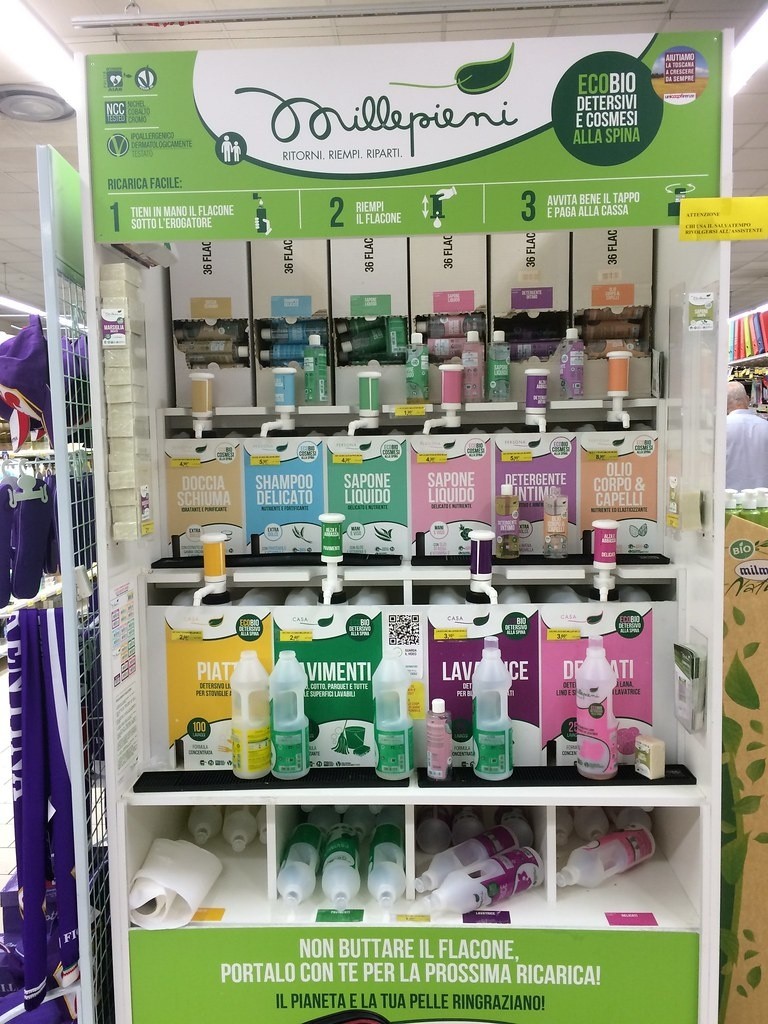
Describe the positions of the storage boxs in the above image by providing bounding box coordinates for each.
[0,846,108,1024]
[635,735,665,779]
[100,263,153,542]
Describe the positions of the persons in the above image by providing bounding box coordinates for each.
[724,381,768,491]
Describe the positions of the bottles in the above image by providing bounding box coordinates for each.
[509,339,561,360]
[175,324,243,341]
[574,806,609,842]
[557,806,573,845]
[426,339,466,356]
[560,329,584,400]
[558,822,655,887]
[222,805,259,851]
[304,334,328,406]
[231,650,270,780]
[496,484,519,558]
[575,635,618,780]
[260,344,308,368]
[188,805,225,844]
[414,804,544,913]
[426,699,452,781]
[417,314,484,338]
[276,804,405,908]
[462,331,485,402]
[336,317,407,364]
[406,333,430,405]
[575,322,643,339]
[543,488,568,559]
[257,806,266,842]
[372,647,414,780]
[485,331,512,401]
[576,307,644,321]
[262,321,328,340]
[178,341,248,363]
[584,340,645,357]
[269,650,310,780]
[472,637,514,781]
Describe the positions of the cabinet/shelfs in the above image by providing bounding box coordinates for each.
[72,26,734,1024]
